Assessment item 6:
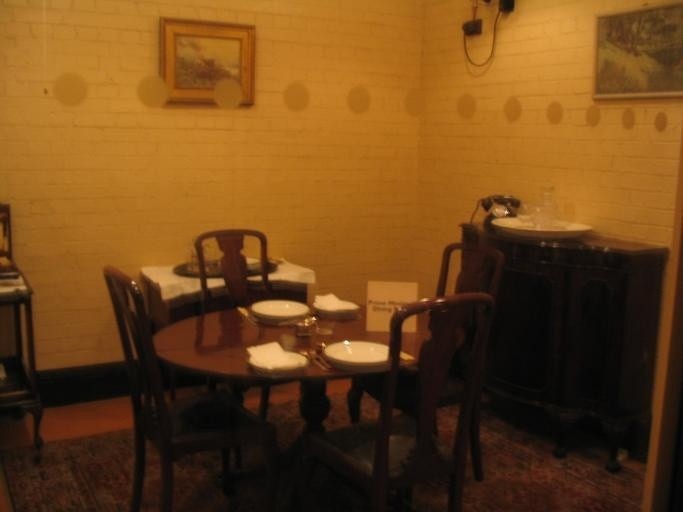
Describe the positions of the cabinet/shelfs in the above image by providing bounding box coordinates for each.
[141,254,320,340]
[457,215,670,475]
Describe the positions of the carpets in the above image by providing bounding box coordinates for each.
[1,374,652,512]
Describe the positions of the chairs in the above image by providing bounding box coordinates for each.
[344,239,505,512]
[192,226,306,472]
[100,265,250,512]
[296,289,499,511]
[1,202,45,451]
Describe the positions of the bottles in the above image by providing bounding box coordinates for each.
[296,314,310,336]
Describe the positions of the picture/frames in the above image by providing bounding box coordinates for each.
[155,15,255,108]
[587,2,683,102]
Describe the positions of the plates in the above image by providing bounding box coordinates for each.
[312,301,360,314]
[247,351,309,376]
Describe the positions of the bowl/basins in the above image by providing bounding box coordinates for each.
[490,216,595,240]
[249,299,310,320]
[323,340,392,373]
